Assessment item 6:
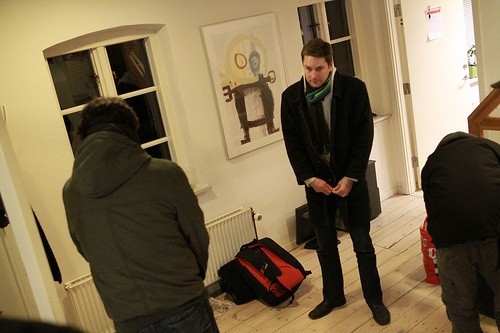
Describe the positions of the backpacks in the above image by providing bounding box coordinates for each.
[236,238,312,306]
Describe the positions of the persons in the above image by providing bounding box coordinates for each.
[60,95,220,333]
[420,129,500,333]
[279,37,392,327]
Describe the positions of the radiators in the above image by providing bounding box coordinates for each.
[64,204,262,333]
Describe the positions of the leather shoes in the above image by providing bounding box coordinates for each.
[308,295,346,319]
[368,301,391,325]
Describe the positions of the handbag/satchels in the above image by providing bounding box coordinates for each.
[419,217,440,285]
[217,259,257,304]
